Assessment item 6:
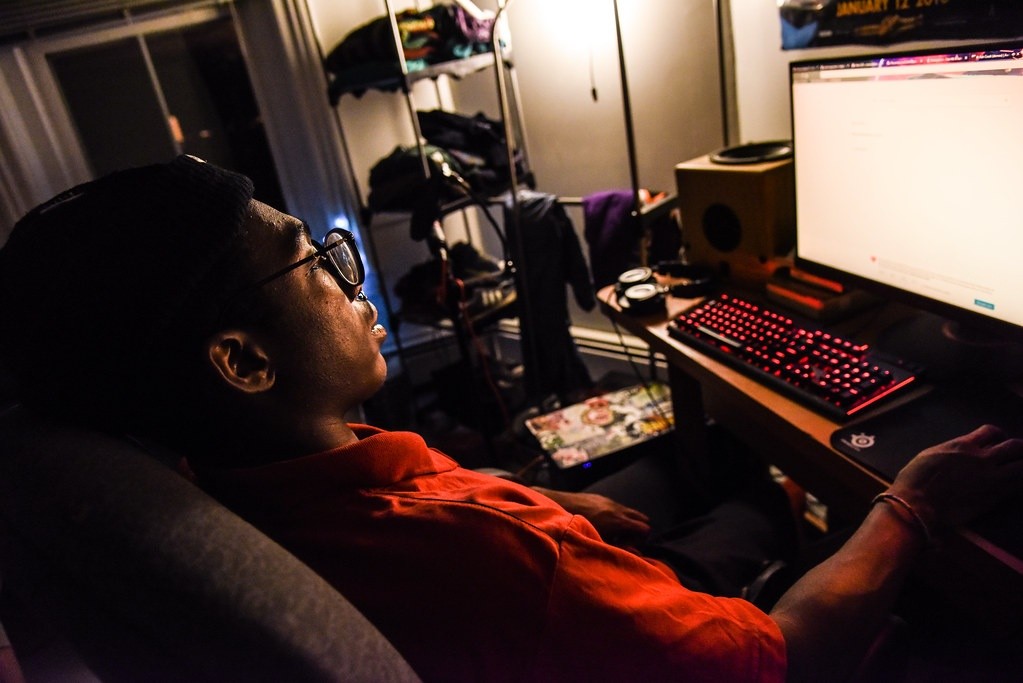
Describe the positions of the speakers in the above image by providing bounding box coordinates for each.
[673,138,794,281]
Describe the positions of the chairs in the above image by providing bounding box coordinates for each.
[1,384,424,682]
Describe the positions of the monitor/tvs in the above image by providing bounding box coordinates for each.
[789,40,1023,387]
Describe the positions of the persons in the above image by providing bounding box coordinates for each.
[0,152,1023,683]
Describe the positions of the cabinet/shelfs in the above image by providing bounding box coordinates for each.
[305,0,540,441]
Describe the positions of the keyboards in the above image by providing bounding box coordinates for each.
[666,290,927,425]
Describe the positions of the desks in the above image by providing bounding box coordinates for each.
[597,270,1023,584]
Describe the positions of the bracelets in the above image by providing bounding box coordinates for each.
[871,490,934,551]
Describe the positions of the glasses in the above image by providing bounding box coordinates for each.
[219,228,365,314]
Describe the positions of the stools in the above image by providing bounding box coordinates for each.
[524,379,676,488]
